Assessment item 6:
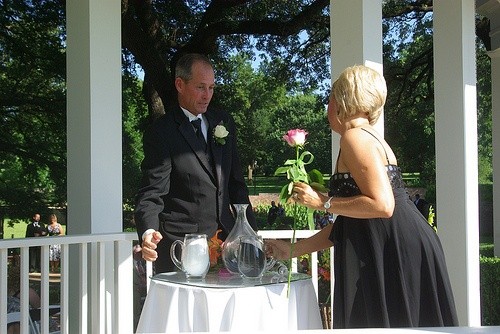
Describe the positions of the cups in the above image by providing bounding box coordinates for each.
[170,234,211,280]
[238,235,276,281]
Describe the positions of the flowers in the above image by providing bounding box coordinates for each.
[214,125,230,139]
[204,229,225,268]
[275,128,324,298]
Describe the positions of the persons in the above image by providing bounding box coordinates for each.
[42,214,63,272]
[264,65,459,329]
[135,53,257,274]
[26,213,45,273]
[268,201,285,225]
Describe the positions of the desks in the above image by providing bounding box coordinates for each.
[135,272,325,333]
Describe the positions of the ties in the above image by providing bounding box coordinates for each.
[190,118,207,154]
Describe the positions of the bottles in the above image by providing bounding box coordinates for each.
[222,204,259,275]
[274,262,288,277]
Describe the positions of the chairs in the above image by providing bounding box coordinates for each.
[6,304,55,333]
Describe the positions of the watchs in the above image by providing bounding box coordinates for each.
[324,196,333,213]
